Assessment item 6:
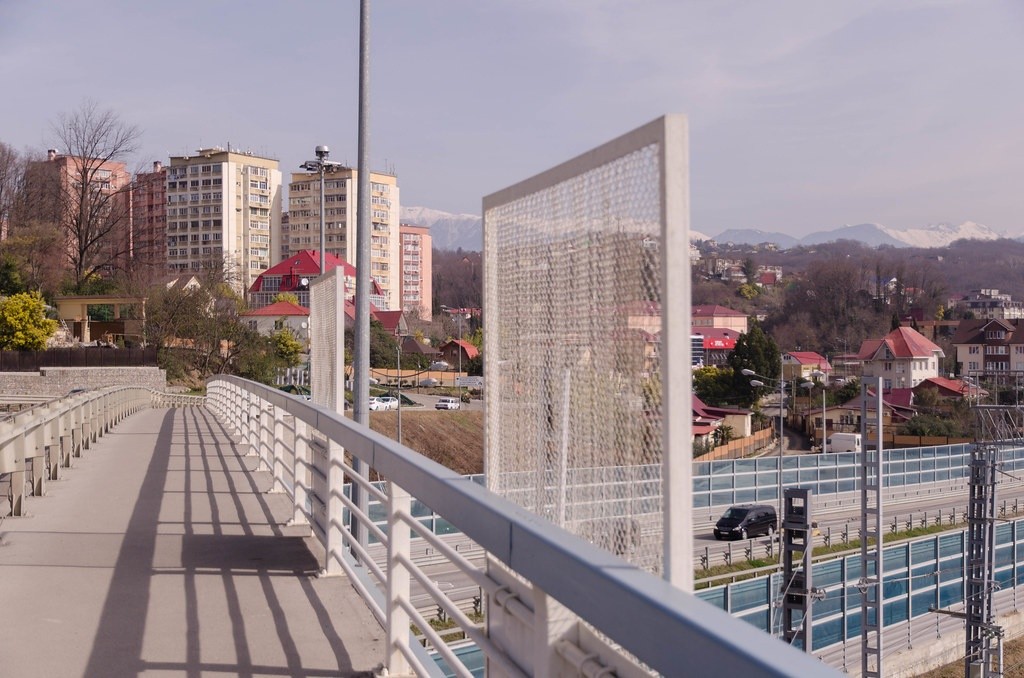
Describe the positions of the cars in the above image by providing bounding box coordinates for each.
[369,396,387,411]
[344,399,350,410]
[381,397,399,410]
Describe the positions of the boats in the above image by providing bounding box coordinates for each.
[420,377,438,387]
[430,362,448,372]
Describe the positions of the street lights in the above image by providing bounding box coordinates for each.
[799,382,815,440]
[740,354,784,566]
[440,304,461,403]
[395,345,401,444]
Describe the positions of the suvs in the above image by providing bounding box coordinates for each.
[435,398,460,410]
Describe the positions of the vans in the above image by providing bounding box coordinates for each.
[819,432,862,453]
[713,504,778,541]
